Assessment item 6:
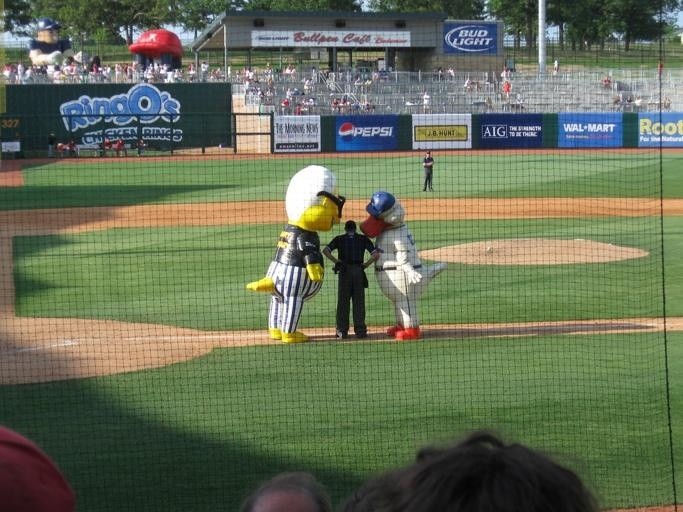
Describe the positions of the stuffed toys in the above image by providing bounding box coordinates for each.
[358,191,450,341]
[244,165,346,343]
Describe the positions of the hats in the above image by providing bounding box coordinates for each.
[345,221,356,230]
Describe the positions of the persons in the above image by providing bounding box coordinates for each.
[239,470,331,512]
[553,57,561,74]
[322,219,380,339]
[3,61,224,83]
[333,430,598,512]
[603,76,611,89]
[448,65,455,81]
[614,94,642,111]
[437,66,443,80]
[422,92,432,115]
[422,150,434,192]
[463,66,514,113]
[228,62,387,115]
